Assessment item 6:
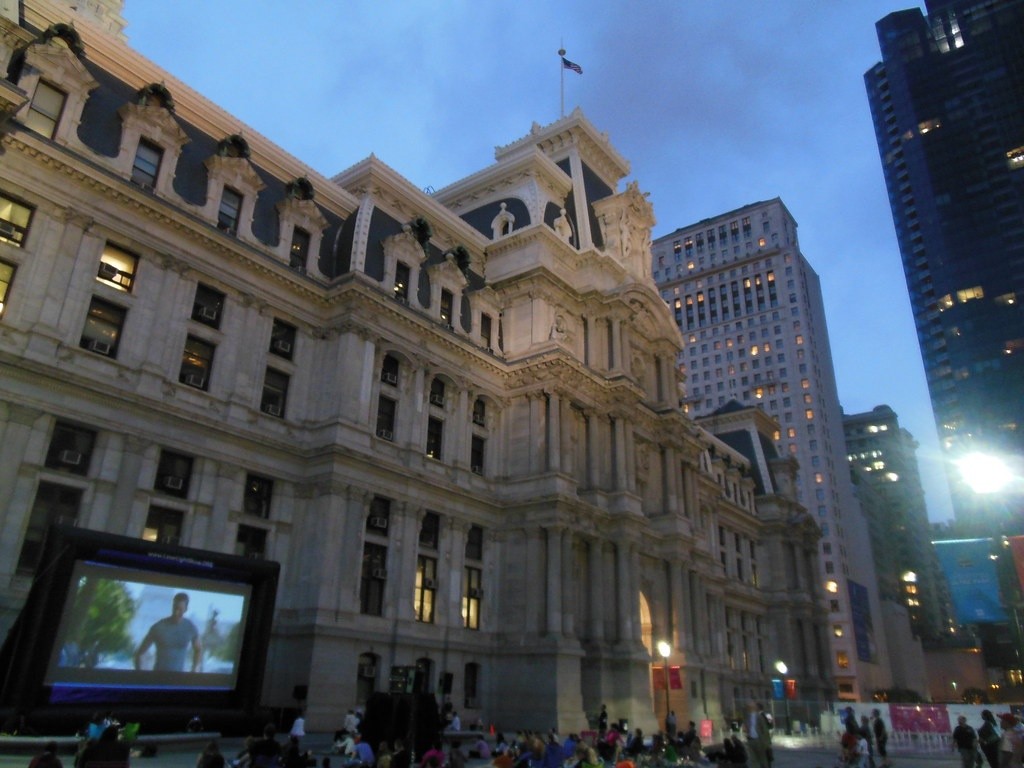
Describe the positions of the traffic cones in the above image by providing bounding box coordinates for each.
[489,724,495,736]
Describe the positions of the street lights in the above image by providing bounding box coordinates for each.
[657,639,670,718]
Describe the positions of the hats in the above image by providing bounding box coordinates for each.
[997,714,1014,723]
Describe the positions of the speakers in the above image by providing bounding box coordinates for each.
[293,684,308,702]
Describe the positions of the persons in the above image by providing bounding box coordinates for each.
[793,691,825,738]
[952,706,1024,768]
[835,704,892,768]
[26,691,787,768]
[135,592,201,687]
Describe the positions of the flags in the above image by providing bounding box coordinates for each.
[563,58,583,75]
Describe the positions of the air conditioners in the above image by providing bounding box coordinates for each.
[0,222,15,238]
[100,263,117,278]
[444,325,454,333]
[88,340,111,355]
[474,415,484,424]
[469,588,483,598]
[294,266,307,275]
[138,183,153,195]
[274,340,290,353]
[185,374,204,388]
[399,299,409,307]
[371,517,387,528]
[359,666,376,678]
[265,404,281,417]
[424,579,438,589]
[58,449,81,466]
[485,348,493,356]
[378,430,391,441]
[382,373,397,384]
[431,394,444,405]
[163,475,183,490]
[199,307,216,320]
[372,569,387,580]
[223,228,236,237]
[472,465,483,475]
